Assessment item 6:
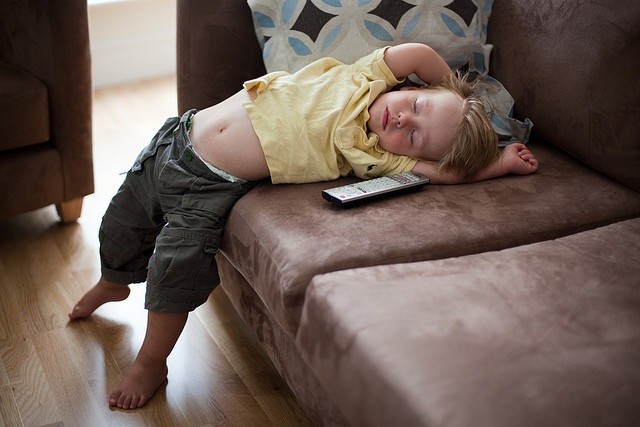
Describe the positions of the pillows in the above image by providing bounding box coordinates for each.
[246,0,534,149]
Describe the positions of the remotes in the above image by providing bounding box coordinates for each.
[321,170,430,207]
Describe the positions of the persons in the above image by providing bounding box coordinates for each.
[69,42,539,410]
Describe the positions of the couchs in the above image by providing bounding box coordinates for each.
[0,0,96,225]
[174,0,640,427]
